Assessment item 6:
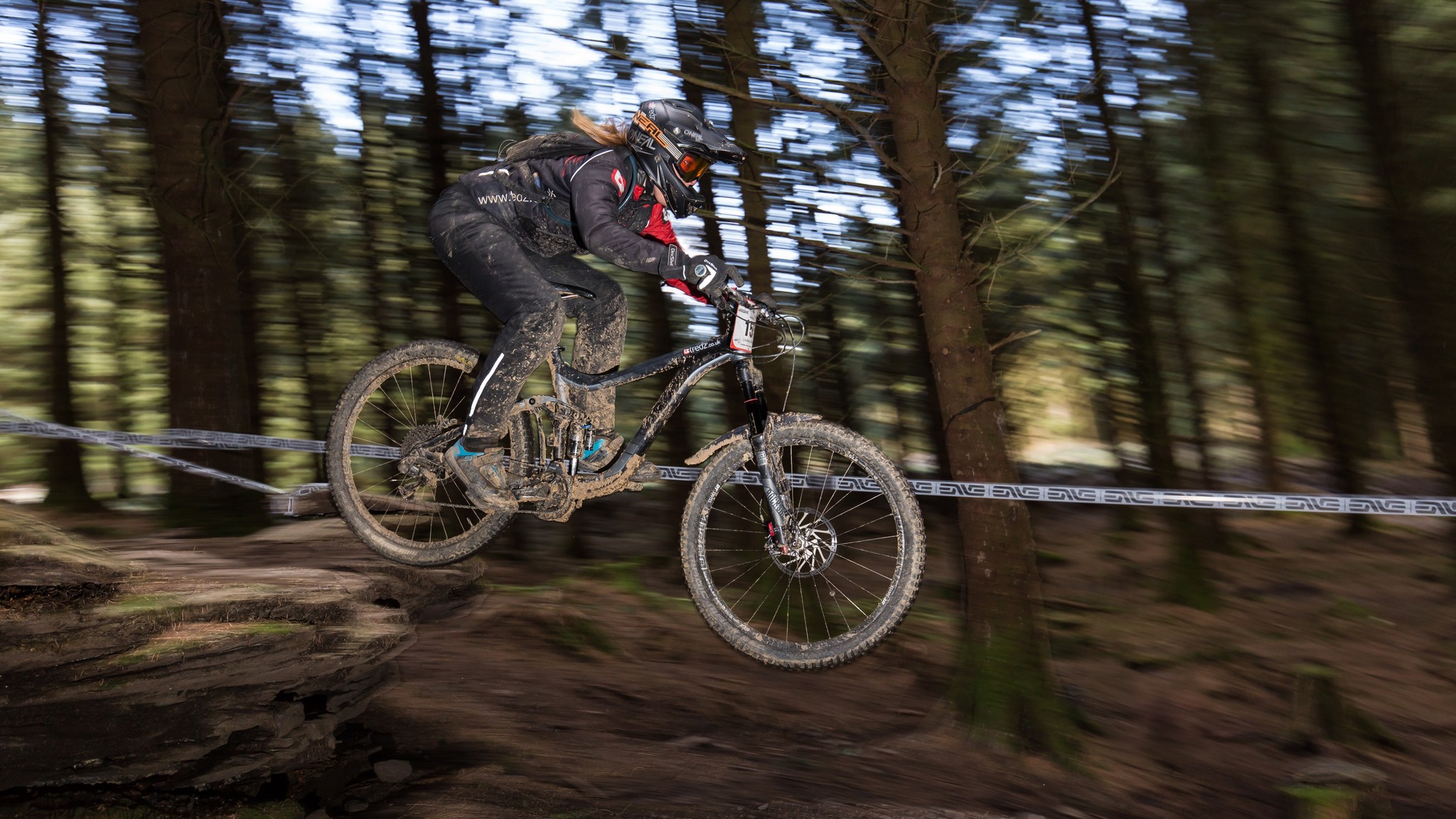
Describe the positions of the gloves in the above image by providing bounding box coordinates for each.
[736,289,777,308]
[658,248,744,296]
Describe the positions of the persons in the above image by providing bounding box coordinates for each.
[429,98,780,514]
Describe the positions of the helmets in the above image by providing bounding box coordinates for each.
[626,99,748,219]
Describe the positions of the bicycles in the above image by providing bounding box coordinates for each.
[326,277,928,671]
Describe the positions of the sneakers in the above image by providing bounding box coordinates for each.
[579,434,662,482]
[445,438,520,513]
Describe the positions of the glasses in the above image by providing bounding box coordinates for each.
[675,152,718,189]
